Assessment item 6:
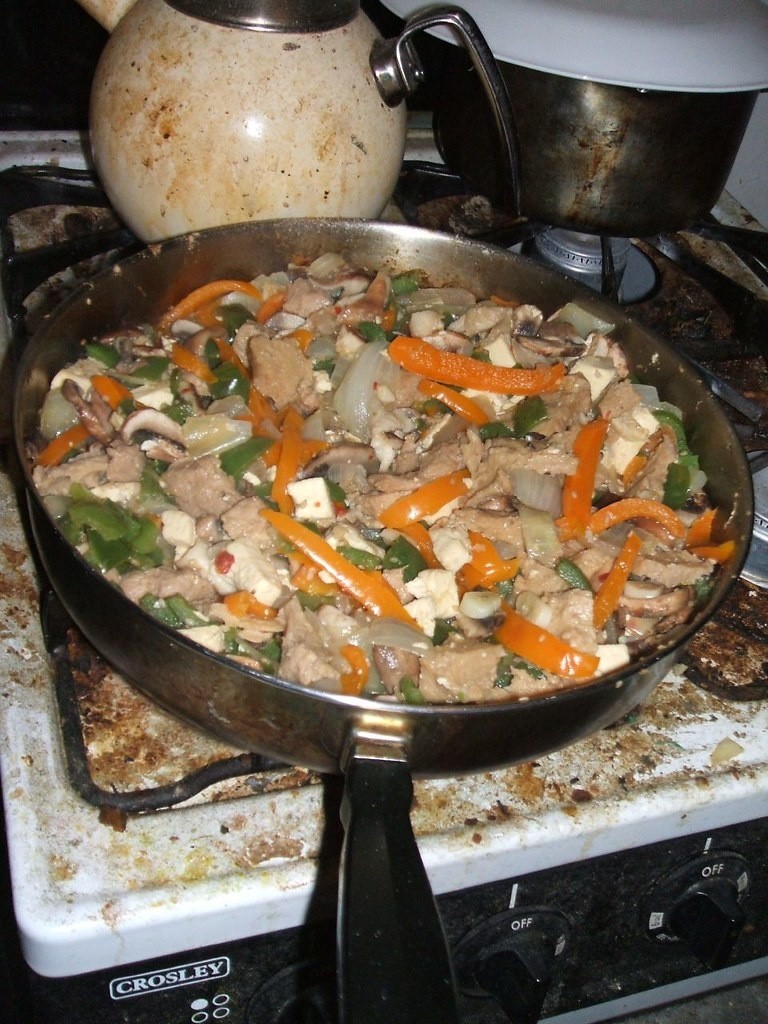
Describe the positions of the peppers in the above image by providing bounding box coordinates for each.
[43,276,735,680]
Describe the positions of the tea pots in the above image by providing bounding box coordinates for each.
[72,0,528,246]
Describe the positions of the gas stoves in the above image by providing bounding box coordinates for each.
[0,112,768,1023]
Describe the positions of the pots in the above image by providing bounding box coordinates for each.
[361,29,768,239]
[0,215,768,1024]
